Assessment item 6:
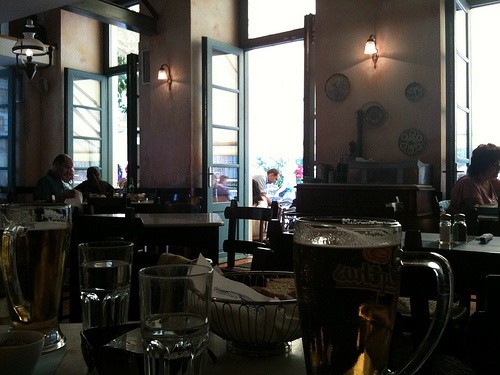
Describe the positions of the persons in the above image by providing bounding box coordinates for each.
[249,178,269,241]
[246,168,281,207]
[210,174,219,201]
[448,141,500,218]
[217,175,234,201]
[31,153,78,206]
[71,166,116,195]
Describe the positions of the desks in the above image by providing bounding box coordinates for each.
[0,324,308,375]
[92,213,224,267]
[397,232,500,311]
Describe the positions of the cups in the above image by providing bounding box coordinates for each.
[0,202,74,352]
[79,240,134,330]
[294,215,454,375]
[138,264,213,375]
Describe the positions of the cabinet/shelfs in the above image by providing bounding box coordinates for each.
[296,183,437,233]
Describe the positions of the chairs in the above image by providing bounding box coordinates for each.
[0,192,500,375]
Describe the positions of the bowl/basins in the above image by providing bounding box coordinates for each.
[0,332,46,375]
[189,271,302,355]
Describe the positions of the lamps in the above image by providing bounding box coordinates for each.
[12,20,48,57]
[363,34,380,69]
[157,64,172,91]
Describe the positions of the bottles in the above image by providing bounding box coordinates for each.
[439,213,451,244]
[452,214,468,244]
